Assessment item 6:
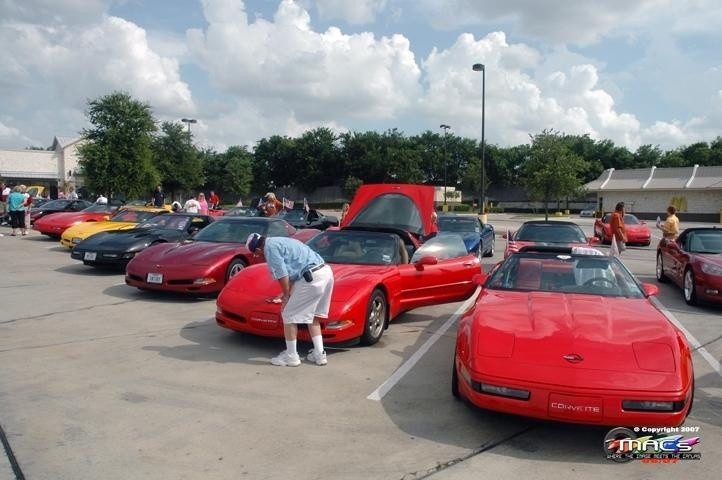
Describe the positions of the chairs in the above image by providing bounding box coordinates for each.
[516,251,541,292]
[572,247,617,287]
[686,235,704,251]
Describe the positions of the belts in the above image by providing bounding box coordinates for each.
[312,264,324,272]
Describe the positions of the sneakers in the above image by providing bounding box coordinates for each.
[270,350,301,366]
[307,351,328,365]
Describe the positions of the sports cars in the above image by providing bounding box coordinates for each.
[451,242,694,433]
[0,186,495,298]
[215,186,484,346]
[501,210,722,306]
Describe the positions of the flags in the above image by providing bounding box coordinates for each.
[304,198,310,212]
[504,230,519,258]
[283,197,294,210]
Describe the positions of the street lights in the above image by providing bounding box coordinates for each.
[183,118,198,138]
[473,62,487,213]
[440,123,451,204]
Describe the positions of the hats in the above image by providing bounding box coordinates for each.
[245,233,262,258]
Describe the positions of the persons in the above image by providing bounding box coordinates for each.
[152,185,220,215]
[264,192,283,216]
[656,206,679,238]
[0,180,108,236]
[246,233,334,365]
[609,202,628,255]
